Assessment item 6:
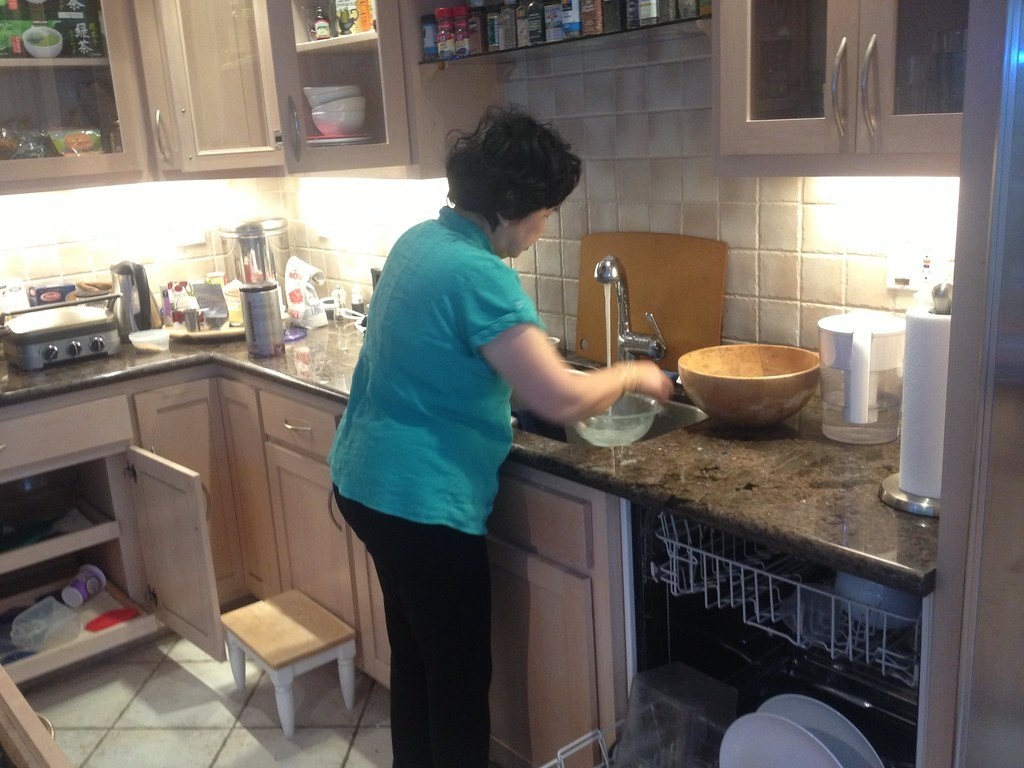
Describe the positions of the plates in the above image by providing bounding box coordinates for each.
[756,694,884,768]
[307,132,373,148]
[719,713,843,768]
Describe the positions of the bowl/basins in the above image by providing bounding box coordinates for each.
[834,570,920,629]
[572,392,662,445]
[678,344,820,429]
[319,297,334,319]
[129,329,170,354]
[22,27,63,59]
[304,84,367,136]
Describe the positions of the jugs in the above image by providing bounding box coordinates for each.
[816,313,906,444]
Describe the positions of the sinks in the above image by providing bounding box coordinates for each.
[509,394,711,448]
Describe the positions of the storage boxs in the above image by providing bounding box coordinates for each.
[0,0,105,58]
[335,0,376,36]
[29,283,76,307]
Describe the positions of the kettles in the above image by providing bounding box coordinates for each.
[109,260,162,343]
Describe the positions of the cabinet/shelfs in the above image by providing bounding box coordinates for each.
[132,0,502,197]
[710,0,967,178]
[0,360,626,768]
[0,0,145,195]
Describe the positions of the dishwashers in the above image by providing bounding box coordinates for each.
[528,499,937,768]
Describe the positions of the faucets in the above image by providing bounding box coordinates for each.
[593,255,668,362]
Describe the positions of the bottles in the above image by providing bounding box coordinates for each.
[332,284,346,322]
[240,281,286,358]
[420,1,714,62]
[315,6,331,40]
[352,286,364,317]
[161,273,243,332]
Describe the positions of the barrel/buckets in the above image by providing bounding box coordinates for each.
[216,216,291,314]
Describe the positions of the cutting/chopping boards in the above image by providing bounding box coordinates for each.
[574,231,728,372]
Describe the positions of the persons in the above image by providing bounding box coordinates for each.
[327,108,673,768]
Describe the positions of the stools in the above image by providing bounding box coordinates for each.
[220,588,358,739]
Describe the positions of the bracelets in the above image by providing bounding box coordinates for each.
[616,361,638,398]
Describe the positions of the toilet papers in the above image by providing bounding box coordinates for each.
[899,306,955,501]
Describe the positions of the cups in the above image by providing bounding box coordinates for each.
[62,565,107,606]
